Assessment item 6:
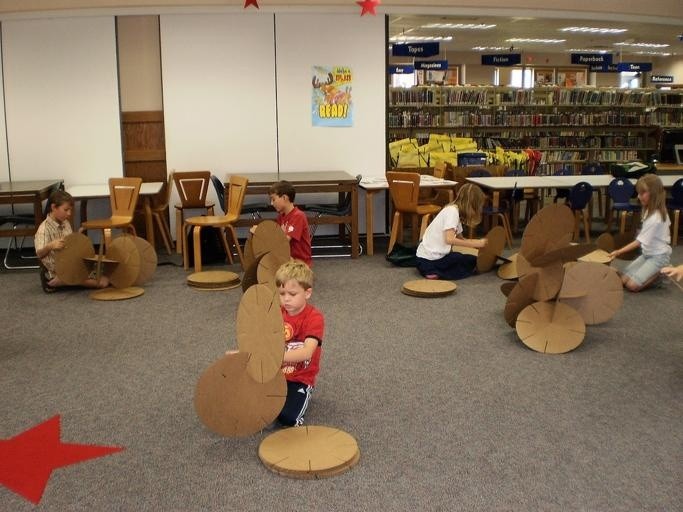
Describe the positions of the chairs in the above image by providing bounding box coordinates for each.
[466,169,514,210]
[580,161,603,217]
[182,175,247,274]
[4,181,66,269]
[133,167,176,259]
[551,169,571,205]
[607,177,643,235]
[385,170,440,257]
[80,178,143,290]
[210,174,269,265]
[501,170,537,235]
[468,208,514,250]
[303,174,364,258]
[418,159,448,204]
[171,170,216,256]
[569,181,594,245]
[662,177,683,246]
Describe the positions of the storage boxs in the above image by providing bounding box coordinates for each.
[452,164,506,202]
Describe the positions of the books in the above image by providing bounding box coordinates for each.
[389,107,683,127]
[389,131,643,148]
[537,163,586,175]
[540,151,638,160]
[390,87,683,104]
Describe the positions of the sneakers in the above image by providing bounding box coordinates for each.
[661,265,673,288]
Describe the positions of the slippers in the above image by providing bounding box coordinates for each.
[40,271,58,293]
[425,273,440,280]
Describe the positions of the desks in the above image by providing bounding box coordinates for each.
[223,170,361,265]
[357,173,458,258]
[66,181,166,248]
[654,162,683,175]
[466,174,683,231]
[0,179,65,239]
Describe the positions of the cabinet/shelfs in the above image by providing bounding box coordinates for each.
[644,89,683,130]
[387,82,664,173]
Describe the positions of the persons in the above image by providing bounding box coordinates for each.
[225,260,324,428]
[250,180,312,268]
[416,185,487,280]
[661,265,683,281]
[607,174,672,291]
[34,191,109,293]
